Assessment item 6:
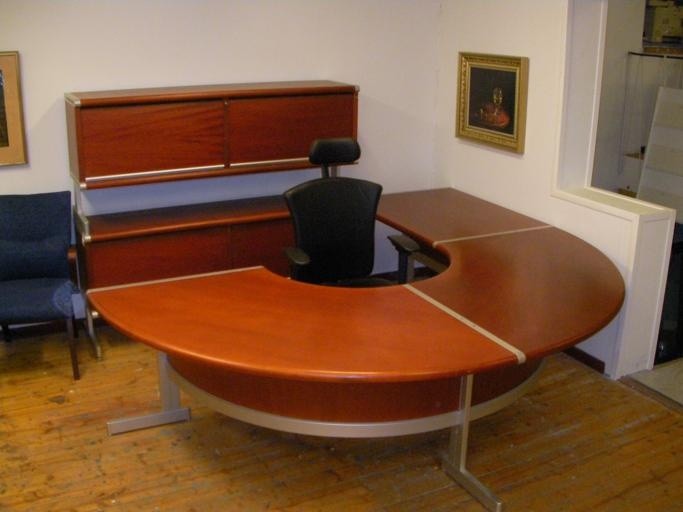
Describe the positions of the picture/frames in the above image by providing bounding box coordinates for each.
[455,51,529,155]
[0,51,29,167]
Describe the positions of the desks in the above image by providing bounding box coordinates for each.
[84,187,626,512]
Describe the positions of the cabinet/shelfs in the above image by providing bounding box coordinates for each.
[64,79,360,361]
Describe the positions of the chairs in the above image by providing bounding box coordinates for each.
[282,138,394,287]
[0,190,81,380]
